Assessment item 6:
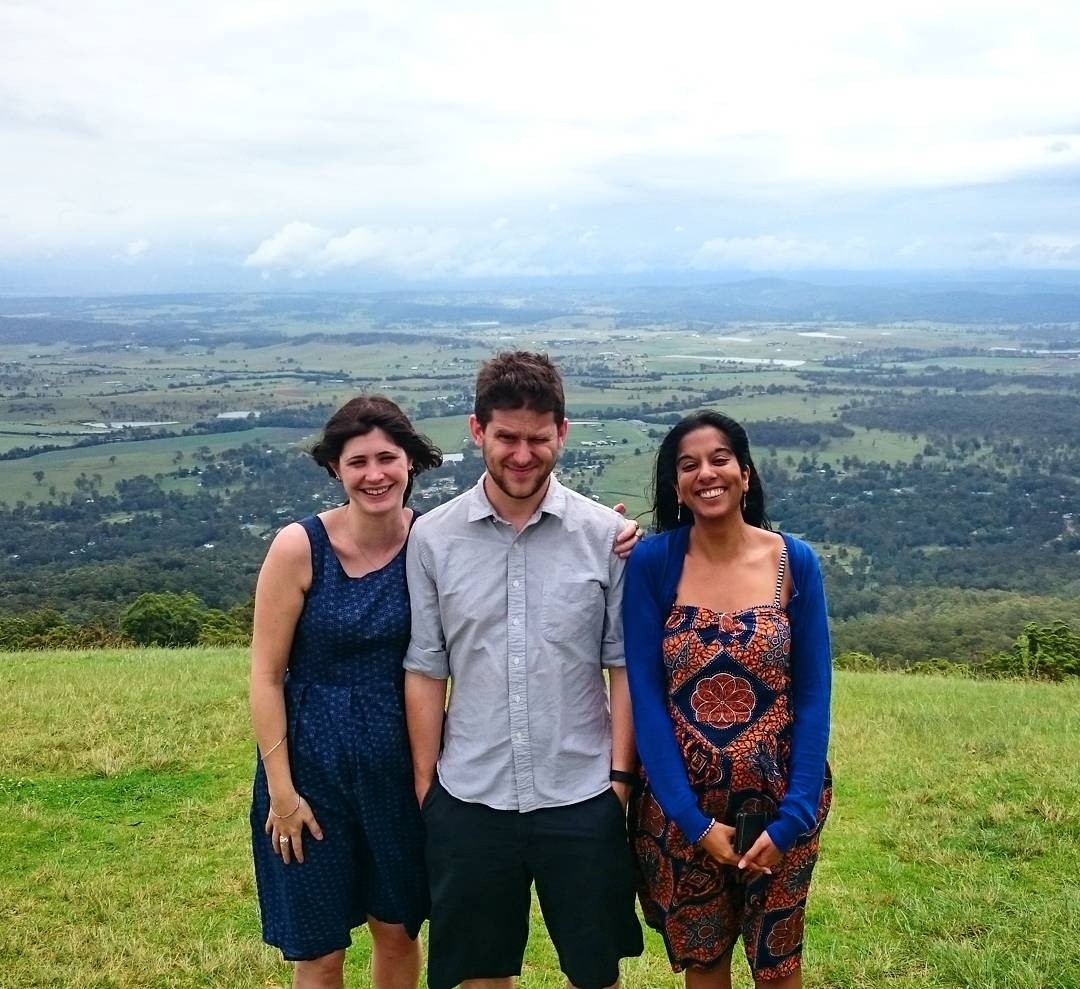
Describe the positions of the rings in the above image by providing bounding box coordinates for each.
[634,521,640,529]
[635,533,641,539]
[279,836,289,848]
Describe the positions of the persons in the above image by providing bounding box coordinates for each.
[249,397,645,989]
[620,411,833,989]
[402,353,645,989]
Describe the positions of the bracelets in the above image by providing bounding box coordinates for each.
[696,817,715,842]
[260,736,287,761]
[610,770,634,786]
[270,794,300,819]
